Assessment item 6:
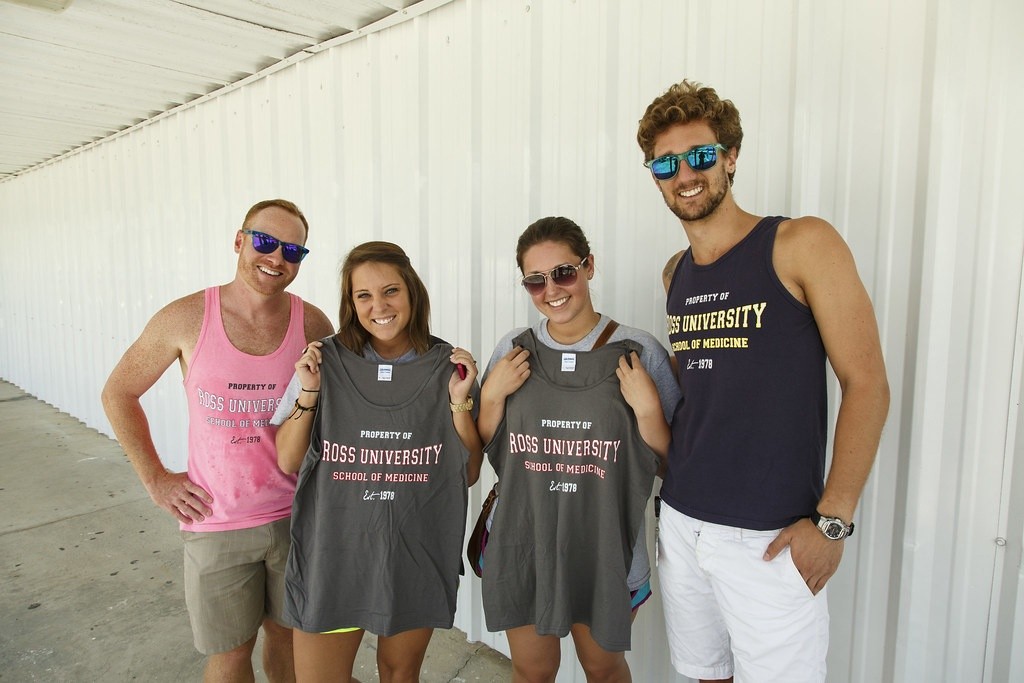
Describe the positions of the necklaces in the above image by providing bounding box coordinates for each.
[373,341,413,365]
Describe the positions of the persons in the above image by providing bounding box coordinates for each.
[268,242,485,683]
[478,217,682,683]
[100,199,334,683]
[638,81,890,683]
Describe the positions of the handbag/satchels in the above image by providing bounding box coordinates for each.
[467,482,499,578]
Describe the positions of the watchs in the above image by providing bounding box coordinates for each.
[449,394,473,412]
[812,510,854,540]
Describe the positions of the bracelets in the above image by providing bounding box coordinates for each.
[302,388,320,392]
[287,398,316,420]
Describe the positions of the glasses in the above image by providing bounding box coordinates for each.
[244,230,310,263]
[644,144,728,180]
[521,256,588,296]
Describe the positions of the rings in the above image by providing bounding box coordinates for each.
[472,361,476,365]
[301,346,309,353]
